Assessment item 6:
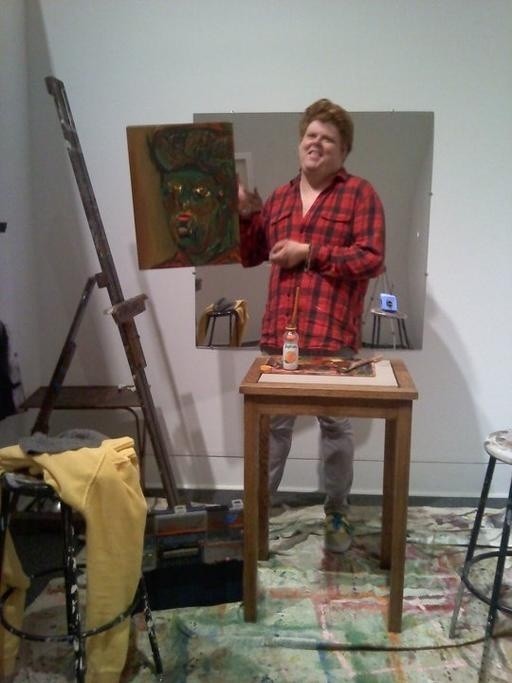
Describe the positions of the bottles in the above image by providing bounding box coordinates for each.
[282,325,300,370]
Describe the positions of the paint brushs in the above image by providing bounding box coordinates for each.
[348,353,384,370]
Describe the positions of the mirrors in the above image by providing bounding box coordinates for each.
[191,109,435,350]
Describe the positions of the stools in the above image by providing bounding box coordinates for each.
[0,467,164,683]
[203,310,241,346]
[446,428,512,682]
[371,307,411,350]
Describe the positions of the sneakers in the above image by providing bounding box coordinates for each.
[323,513,352,553]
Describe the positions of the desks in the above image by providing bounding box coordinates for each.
[238,356,418,634]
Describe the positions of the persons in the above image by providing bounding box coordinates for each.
[235,99,385,551]
